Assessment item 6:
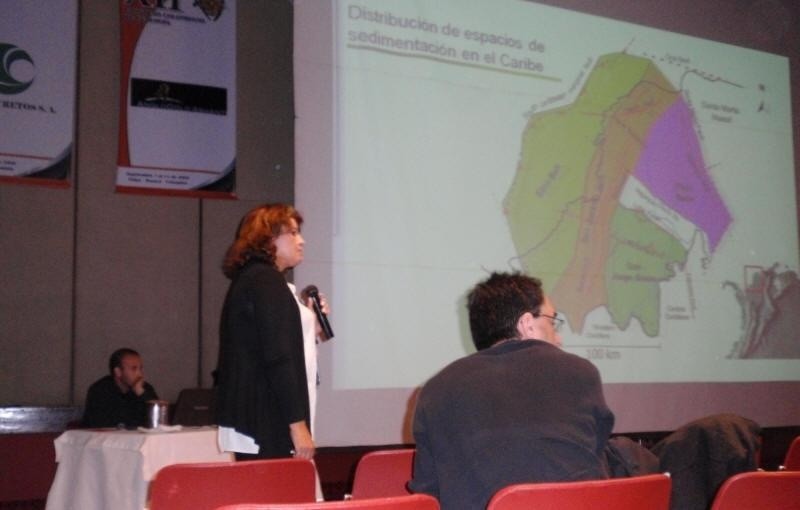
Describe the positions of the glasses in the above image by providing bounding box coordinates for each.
[539,313,567,333]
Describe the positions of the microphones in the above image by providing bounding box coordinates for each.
[306,284,334,341]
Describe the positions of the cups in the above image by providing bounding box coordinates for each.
[146,399,170,429]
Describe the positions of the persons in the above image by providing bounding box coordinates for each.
[80,348,160,429]
[405,270,616,507]
[206,200,330,460]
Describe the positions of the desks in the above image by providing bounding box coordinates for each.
[56,427,237,510]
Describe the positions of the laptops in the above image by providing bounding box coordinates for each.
[169,388,217,426]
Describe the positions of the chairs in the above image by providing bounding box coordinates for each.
[146,449,440,507]
[484,475,675,508]
[711,437,799,509]
